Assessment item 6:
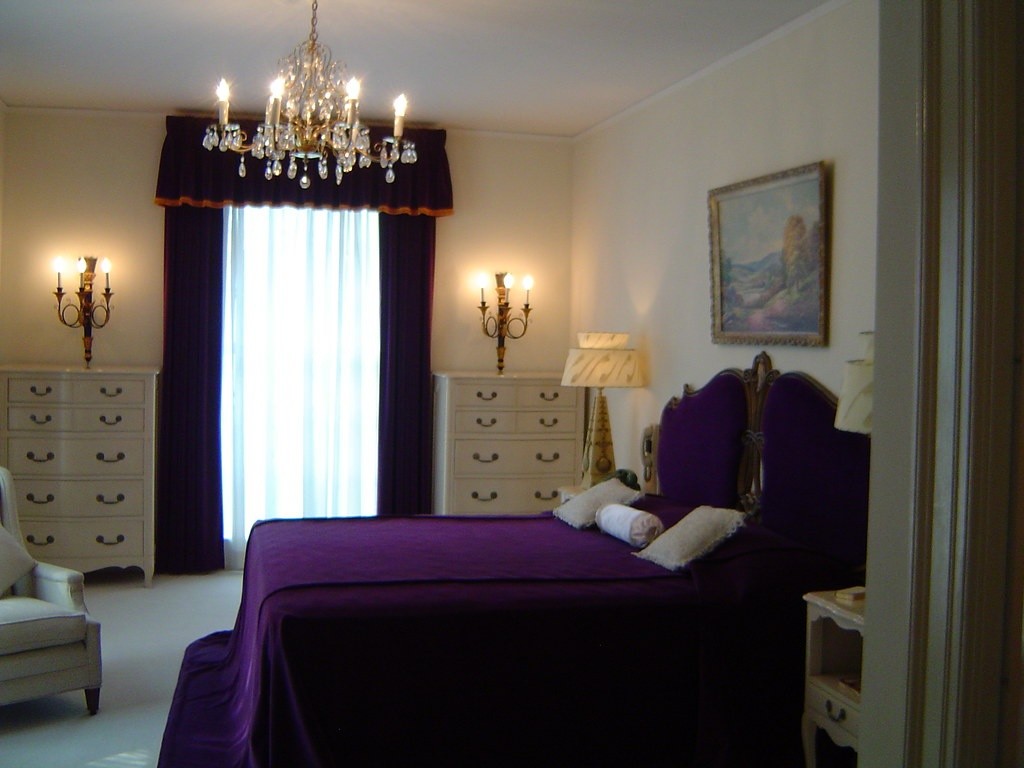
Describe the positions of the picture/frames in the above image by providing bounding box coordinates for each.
[707,160,828,348]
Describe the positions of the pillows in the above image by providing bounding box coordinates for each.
[630,505,747,572]
[552,478,645,529]
[0,522,37,598]
[595,502,664,548]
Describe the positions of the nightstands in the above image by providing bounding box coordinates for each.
[801,590,867,768]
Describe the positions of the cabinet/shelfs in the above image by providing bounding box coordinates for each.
[0,364,163,589]
[432,368,585,515]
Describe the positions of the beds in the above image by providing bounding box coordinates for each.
[156,352,871,768]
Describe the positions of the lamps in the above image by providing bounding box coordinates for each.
[560,332,648,491]
[473,271,533,374]
[834,331,874,435]
[52,256,113,370]
[202,0,417,190]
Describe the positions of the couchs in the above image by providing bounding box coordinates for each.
[0,466,103,715]
